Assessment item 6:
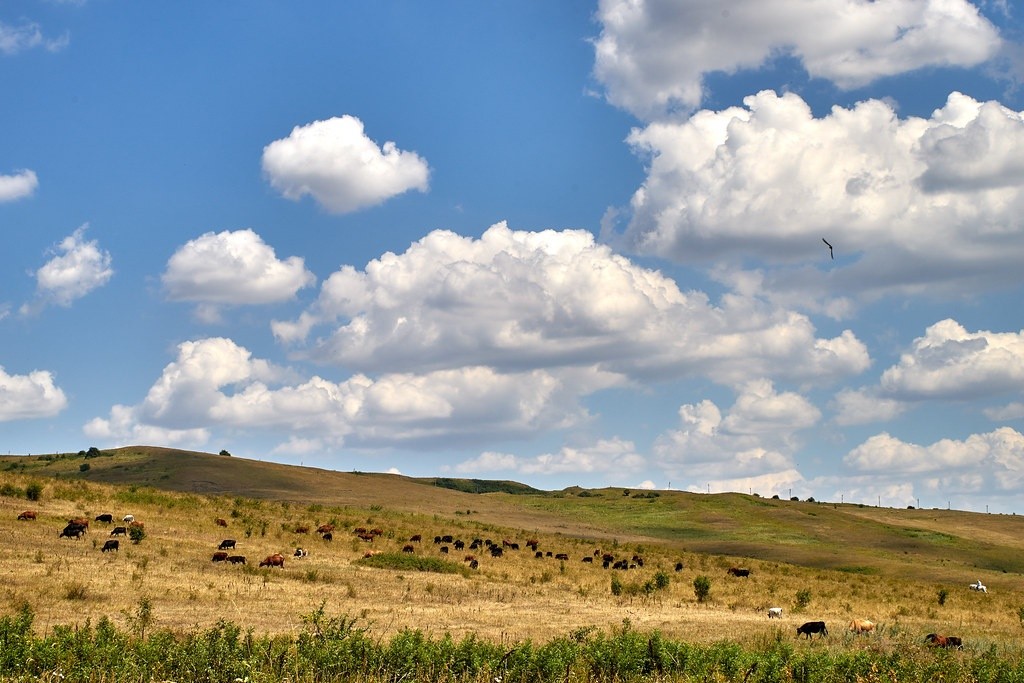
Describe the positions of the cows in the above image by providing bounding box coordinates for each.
[434,535,519,569]
[924,633,963,652]
[767,608,783,619]
[727,568,753,579]
[212,552,247,565]
[60,514,144,553]
[292,524,335,541]
[970,580,987,594]
[401,534,421,553]
[259,554,285,570]
[849,619,874,637]
[351,528,383,542]
[294,548,308,558]
[218,540,237,550]
[526,538,644,570]
[18,511,36,522]
[217,519,229,527]
[361,551,375,558]
[675,563,682,572]
[797,621,828,639]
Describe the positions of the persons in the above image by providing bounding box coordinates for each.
[976,580,981,591]
